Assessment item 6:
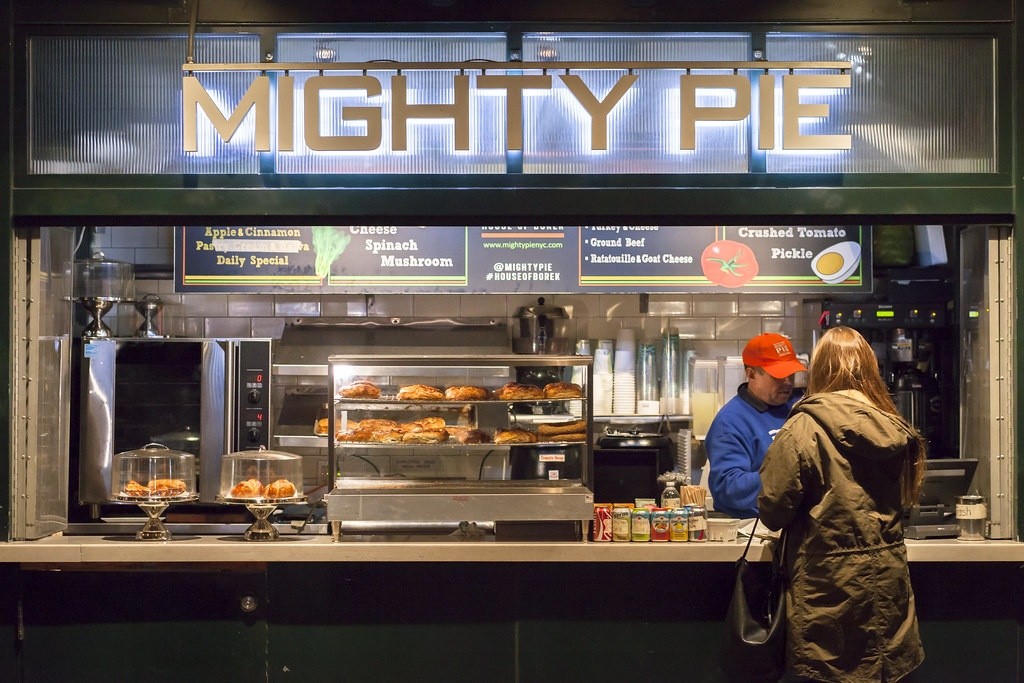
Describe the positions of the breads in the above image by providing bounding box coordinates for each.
[122,479,186,496]
[315,378,589,445]
[230,478,296,498]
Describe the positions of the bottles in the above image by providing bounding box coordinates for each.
[661,481,680,508]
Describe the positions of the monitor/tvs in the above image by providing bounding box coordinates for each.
[917,458,979,511]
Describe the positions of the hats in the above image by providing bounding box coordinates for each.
[741,332,808,378]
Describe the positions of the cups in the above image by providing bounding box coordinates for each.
[955,496,986,540]
[593,339,614,416]
[613,329,636,415]
[569,340,591,416]
[691,505,708,520]
[660,327,690,416]
[635,337,660,415]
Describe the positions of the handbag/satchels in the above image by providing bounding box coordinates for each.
[717,517,788,683]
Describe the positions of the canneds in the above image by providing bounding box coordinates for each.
[592,505,708,542]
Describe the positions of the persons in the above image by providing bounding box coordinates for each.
[758,325,927,683]
[706,334,808,518]
